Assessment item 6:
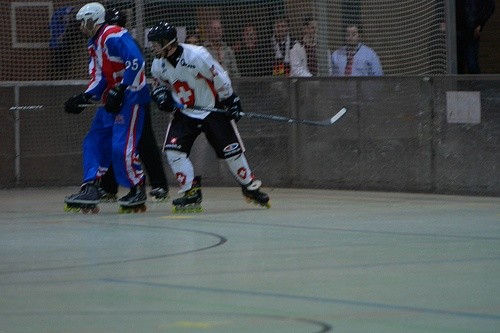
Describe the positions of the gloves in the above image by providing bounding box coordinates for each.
[222,91,242,122]
[64,93,92,114]
[151,86,174,113]
[103,86,125,114]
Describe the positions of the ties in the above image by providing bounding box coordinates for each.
[344,48,355,76]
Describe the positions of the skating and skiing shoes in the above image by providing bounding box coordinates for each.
[64,182,99,214]
[149,187,171,202]
[98,188,116,203]
[172,188,204,213]
[241,178,271,209]
[117,184,147,214]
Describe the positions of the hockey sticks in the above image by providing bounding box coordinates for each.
[176,103,347,127]
[1,103,122,111]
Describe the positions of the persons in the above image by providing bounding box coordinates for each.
[430,0,497,74]
[94,7,169,203]
[332,23,384,76]
[185,16,331,76]
[147,22,272,214]
[63,2,146,214]
[51,0,86,79]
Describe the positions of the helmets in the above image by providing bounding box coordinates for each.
[147,21,177,52]
[105,8,126,28]
[75,2,106,34]
